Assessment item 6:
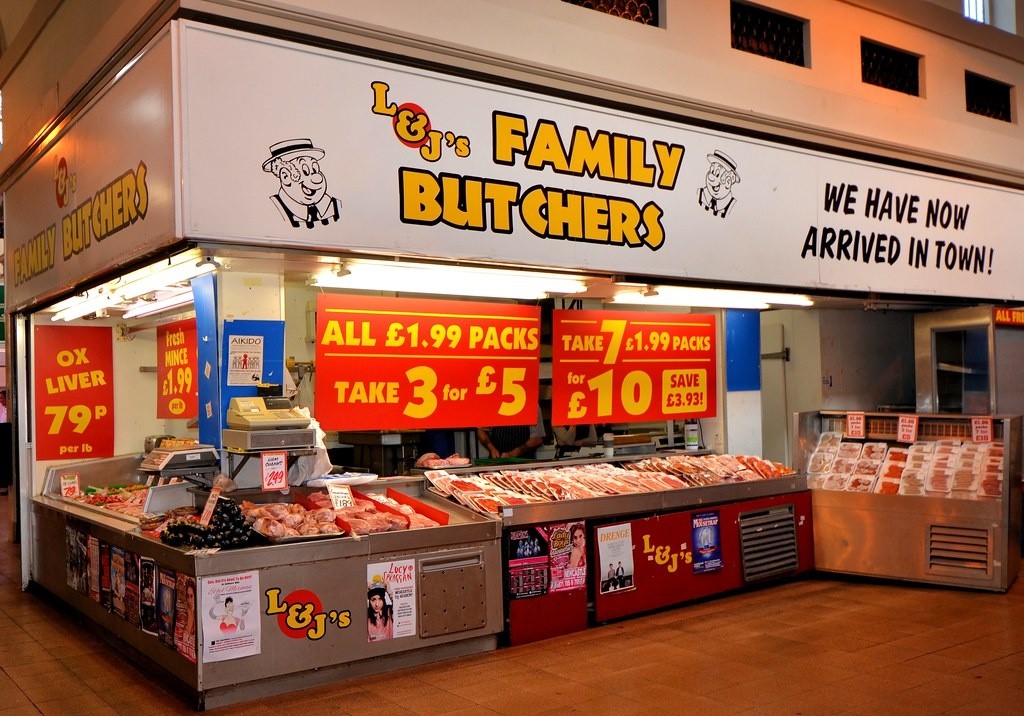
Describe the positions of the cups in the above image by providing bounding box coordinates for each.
[685,422,698,450]
[603,433,615,457]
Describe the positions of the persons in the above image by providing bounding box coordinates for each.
[603,561,624,592]
[552,424,597,459]
[368,579,394,643]
[114,571,124,601]
[562,523,586,569]
[70,550,86,591]
[142,582,154,606]
[478,404,546,458]
[179,578,195,636]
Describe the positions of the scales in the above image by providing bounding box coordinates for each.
[140,446,220,470]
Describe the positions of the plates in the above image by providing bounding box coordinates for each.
[806,431,1004,498]
[425,451,796,514]
[417,464,472,469]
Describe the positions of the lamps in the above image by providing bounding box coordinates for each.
[306,259,592,300]
[863,305,923,311]
[602,287,816,309]
[51,256,220,322]
